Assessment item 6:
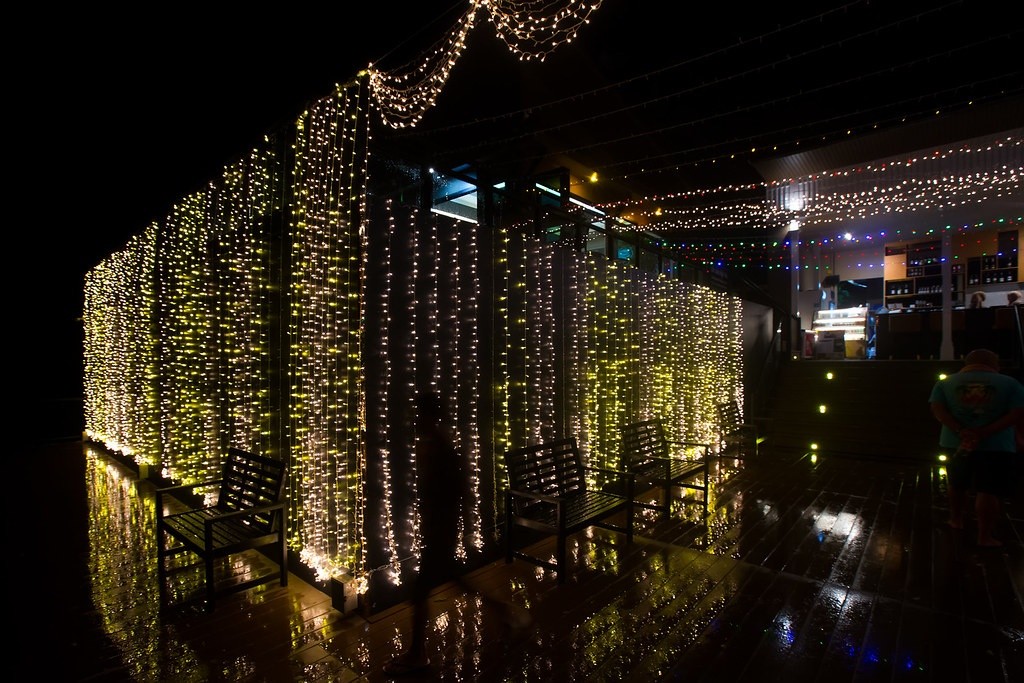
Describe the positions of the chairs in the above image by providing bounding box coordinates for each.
[155,445,290,615]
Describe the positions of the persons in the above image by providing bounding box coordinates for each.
[930,349,1023,546]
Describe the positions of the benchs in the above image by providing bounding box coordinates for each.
[503,436,634,582]
[716,401,759,463]
[619,417,710,522]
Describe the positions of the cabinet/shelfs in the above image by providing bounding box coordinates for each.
[813,306,868,343]
[883,225,1024,314]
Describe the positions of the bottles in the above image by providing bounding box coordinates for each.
[888,257,942,313]
[968,256,1015,285]
